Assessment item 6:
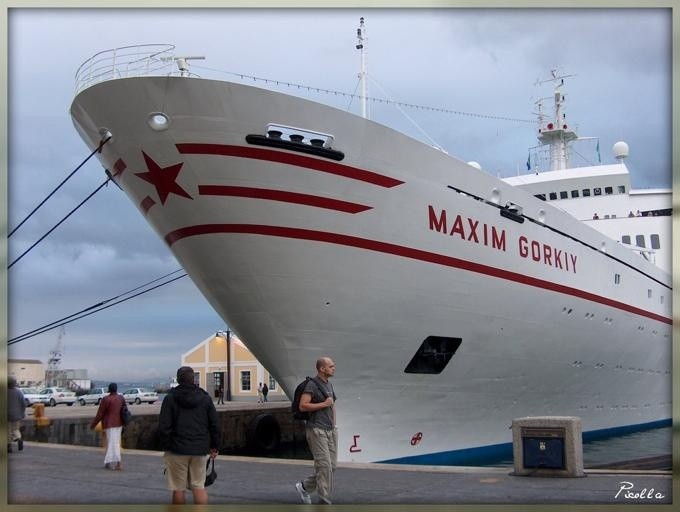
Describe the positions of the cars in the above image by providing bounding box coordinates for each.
[12,385,162,406]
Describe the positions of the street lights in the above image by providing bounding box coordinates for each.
[214,328,236,402]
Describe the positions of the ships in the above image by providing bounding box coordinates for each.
[63,14,674,467]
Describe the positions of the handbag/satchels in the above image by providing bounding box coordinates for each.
[121,407,132,425]
[205,472,216,486]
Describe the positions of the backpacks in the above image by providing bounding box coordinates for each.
[292,375,327,420]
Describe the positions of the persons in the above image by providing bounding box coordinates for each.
[7,375,27,457]
[155,365,220,512]
[257,381,264,405]
[290,357,340,506]
[89,381,133,473]
[216,382,225,405]
[263,383,269,403]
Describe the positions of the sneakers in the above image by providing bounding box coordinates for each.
[217,402,224,404]
[104,461,124,470]
[296,482,312,504]
[18,438,23,450]
[258,400,268,403]
[7,443,12,453]
